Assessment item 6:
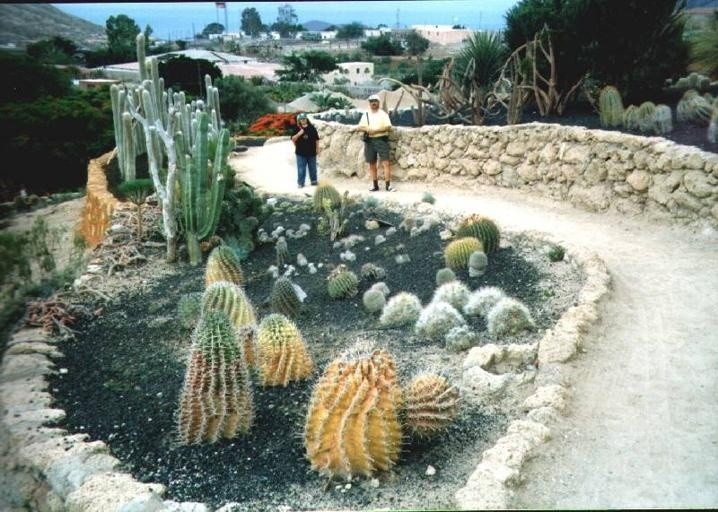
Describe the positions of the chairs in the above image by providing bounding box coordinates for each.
[368,186,380,192]
[386,185,397,192]
[297,180,320,188]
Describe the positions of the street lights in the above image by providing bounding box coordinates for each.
[360,131,370,144]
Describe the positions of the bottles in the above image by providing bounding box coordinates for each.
[367,94,381,102]
[298,113,308,121]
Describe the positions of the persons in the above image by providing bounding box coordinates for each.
[290,113,321,189]
[356,94,397,193]
